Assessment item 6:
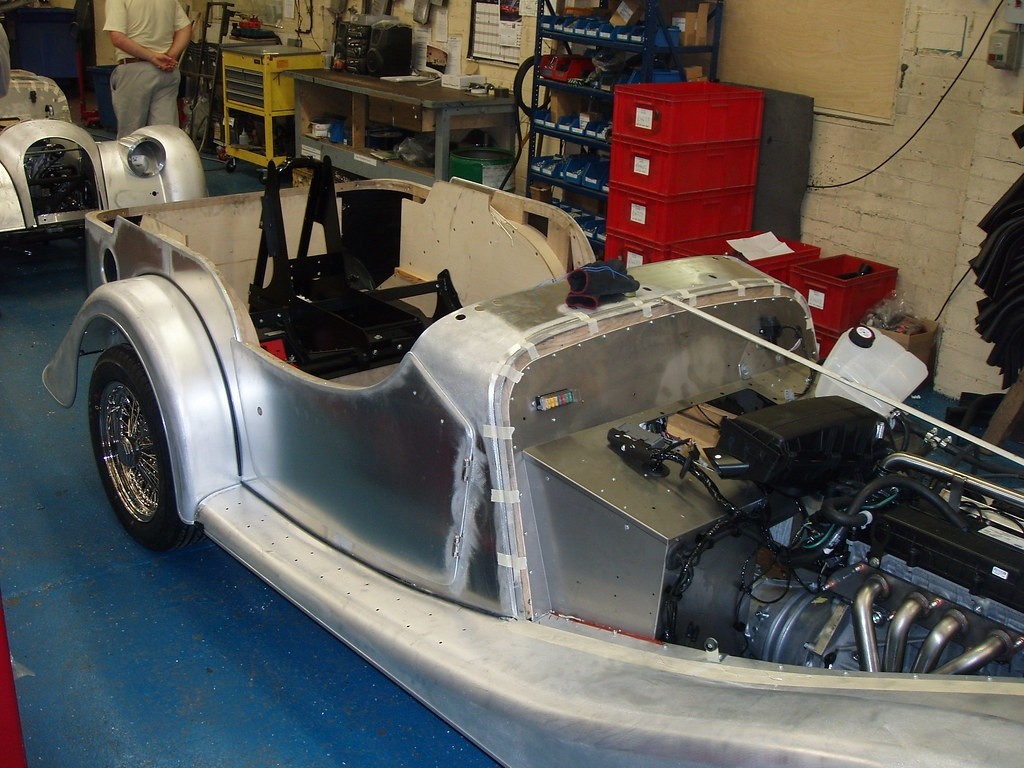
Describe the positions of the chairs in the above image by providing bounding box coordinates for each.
[248,154,461,377]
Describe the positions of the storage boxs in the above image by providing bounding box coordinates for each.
[603,81,942,386]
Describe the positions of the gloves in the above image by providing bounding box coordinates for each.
[566,258,640,310]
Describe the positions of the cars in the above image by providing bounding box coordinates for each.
[0,68,210,268]
[40,156,1024,767]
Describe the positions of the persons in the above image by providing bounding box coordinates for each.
[0,24,12,99]
[102,0,192,141]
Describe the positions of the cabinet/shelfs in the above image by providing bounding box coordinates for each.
[284,66,515,187]
[526,0,729,259]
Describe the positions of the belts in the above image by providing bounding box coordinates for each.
[119,57,143,65]
